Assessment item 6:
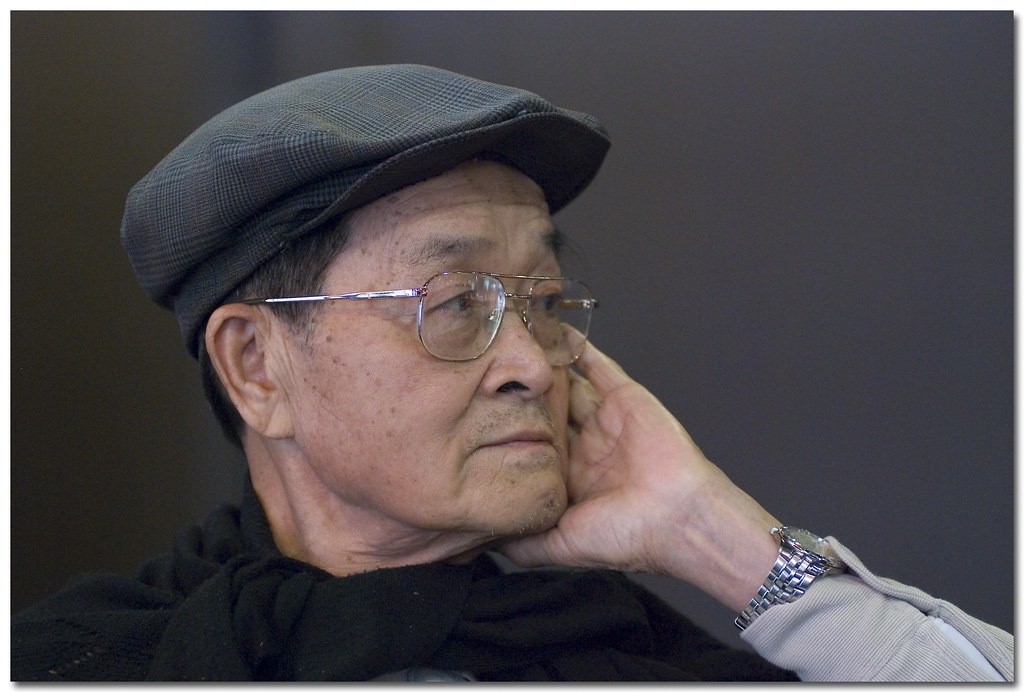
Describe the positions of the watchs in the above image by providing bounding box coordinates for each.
[733,526,846,632]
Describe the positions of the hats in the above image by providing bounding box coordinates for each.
[119,63,613,361]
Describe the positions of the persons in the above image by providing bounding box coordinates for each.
[11,63,1013,681]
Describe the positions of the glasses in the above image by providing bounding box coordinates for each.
[240,271,600,366]
